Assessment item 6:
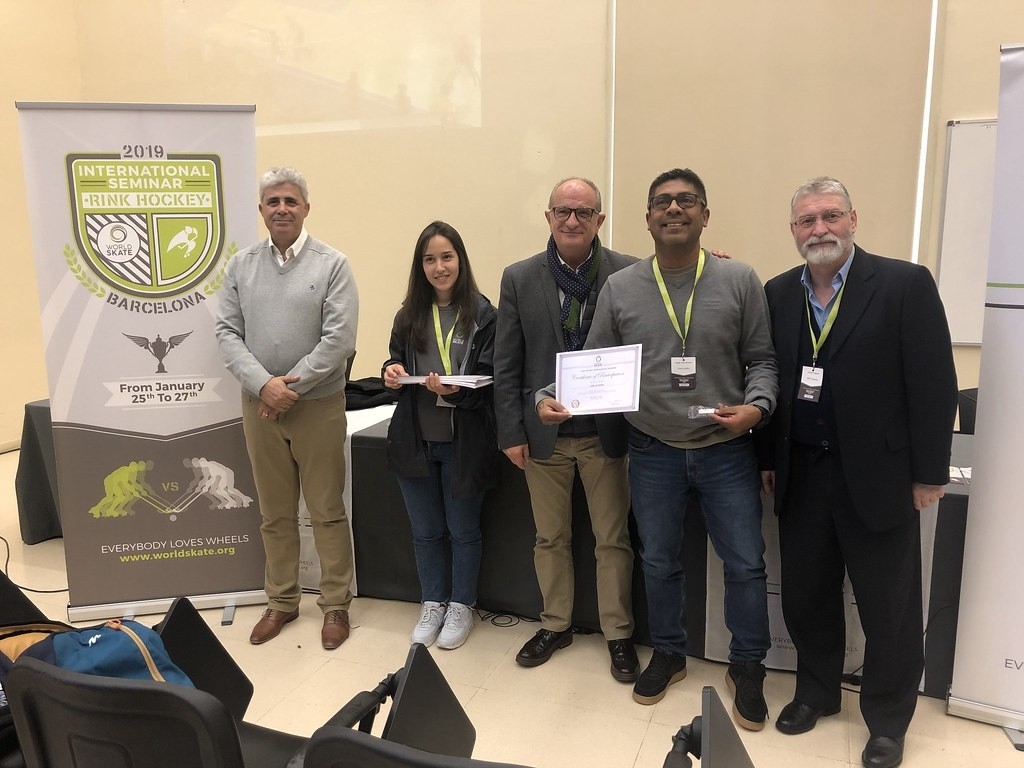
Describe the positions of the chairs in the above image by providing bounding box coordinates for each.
[0,596,755,768]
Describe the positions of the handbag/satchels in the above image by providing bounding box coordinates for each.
[16,617,195,690]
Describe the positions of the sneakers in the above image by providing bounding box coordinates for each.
[633,649,686,705]
[725,664,770,731]
[437,603,473,649]
[412,601,445,647]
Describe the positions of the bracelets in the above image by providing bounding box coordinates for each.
[754,405,770,430]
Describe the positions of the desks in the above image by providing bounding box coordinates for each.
[350,414,972,699]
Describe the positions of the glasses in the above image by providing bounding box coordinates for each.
[549,205,600,223]
[649,194,705,211]
[792,210,851,229]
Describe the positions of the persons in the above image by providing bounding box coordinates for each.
[214,167,359,649]
[752,177,958,768]
[536,168,780,732]
[492,178,730,682]
[380,222,498,651]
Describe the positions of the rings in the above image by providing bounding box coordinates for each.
[263,412,269,417]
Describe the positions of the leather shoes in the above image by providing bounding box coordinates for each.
[515,627,573,666]
[250,606,300,644]
[862,734,904,768]
[322,609,359,649]
[607,638,640,681]
[776,696,841,735]
